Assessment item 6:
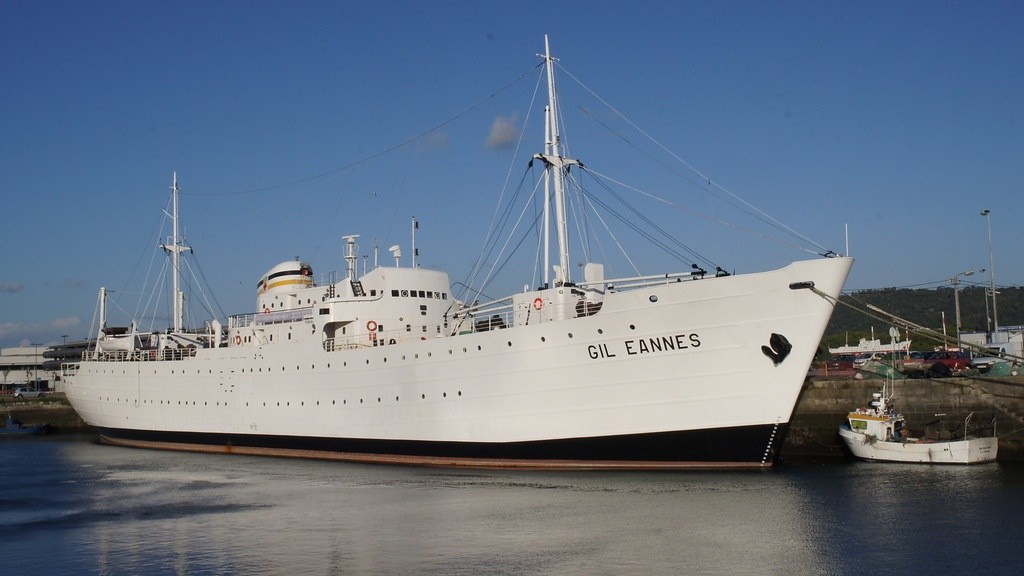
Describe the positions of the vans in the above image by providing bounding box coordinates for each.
[971,342,1018,372]
[852,352,887,369]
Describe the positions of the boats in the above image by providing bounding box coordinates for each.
[0,410,46,435]
[62,35,856,468]
[823,326,912,354]
[839,372,998,464]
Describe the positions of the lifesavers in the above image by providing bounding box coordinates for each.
[367,321,377,331]
[236,335,241,344]
[265,308,270,314]
[534,298,543,310]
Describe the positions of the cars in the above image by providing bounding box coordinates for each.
[882,349,967,368]
[824,354,855,370]
[13,387,46,398]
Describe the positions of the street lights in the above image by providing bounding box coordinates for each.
[980,209,998,335]
[953,271,975,347]
